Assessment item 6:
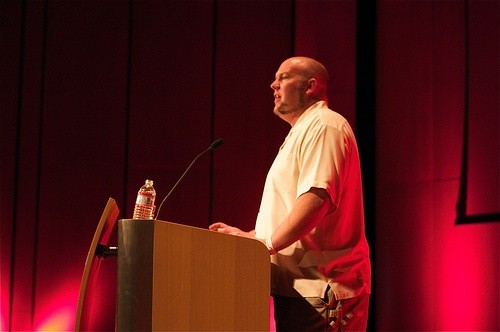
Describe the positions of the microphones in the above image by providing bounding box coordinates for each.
[155,139,224,221]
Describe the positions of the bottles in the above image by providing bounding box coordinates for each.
[133,180,156,220]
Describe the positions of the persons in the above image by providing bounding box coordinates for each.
[209,56,371,332]
